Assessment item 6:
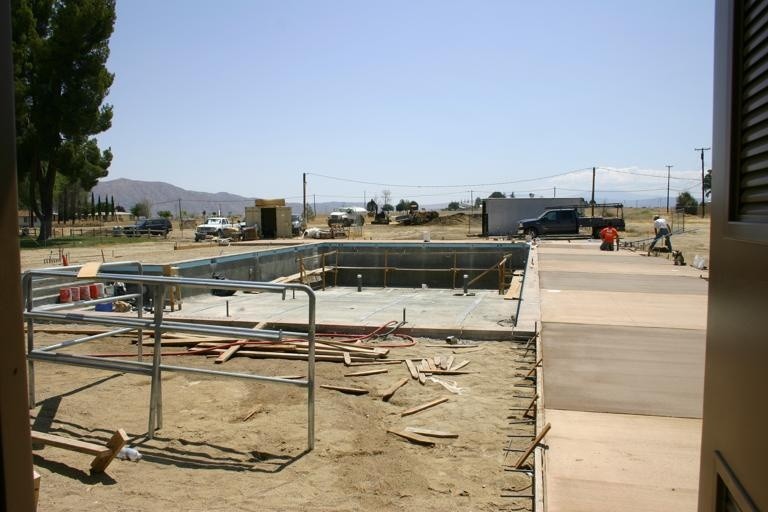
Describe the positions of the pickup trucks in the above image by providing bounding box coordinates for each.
[518,204,625,241]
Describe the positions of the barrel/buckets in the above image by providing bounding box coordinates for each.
[94,283,104,298]
[71,287,80,301]
[80,286,90,300]
[114,285,124,296]
[90,284,98,298]
[60,288,71,302]
[106,287,114,297]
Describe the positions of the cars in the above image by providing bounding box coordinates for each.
[292,214,307,236]
[195,217,246,236]
[123,218,172,236]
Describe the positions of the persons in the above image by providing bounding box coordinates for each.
[599,222,619,251]
[648,215,674,256]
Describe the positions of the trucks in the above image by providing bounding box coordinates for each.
[328,208,368,227]
